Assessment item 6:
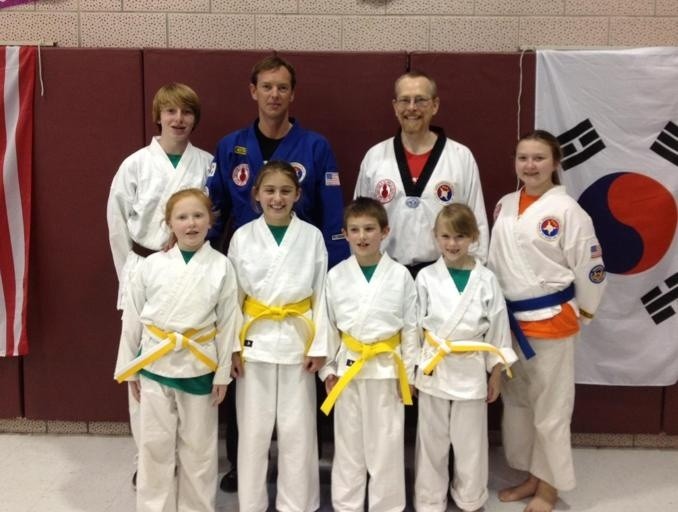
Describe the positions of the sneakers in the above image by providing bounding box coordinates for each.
[220,469,237,492]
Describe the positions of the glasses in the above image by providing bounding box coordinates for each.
[396,99,434,107]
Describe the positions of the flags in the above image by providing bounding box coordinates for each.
[0,41,38,362]
[532,43,676,386]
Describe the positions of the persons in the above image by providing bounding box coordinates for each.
[408,201,517,512]
[207,51,350,492]
[105,79,216,494]
[350,68,492,277]
[113,186,238,512]
[227,163,330,512]
[486,129,607,512]
[314,194,421,512]
